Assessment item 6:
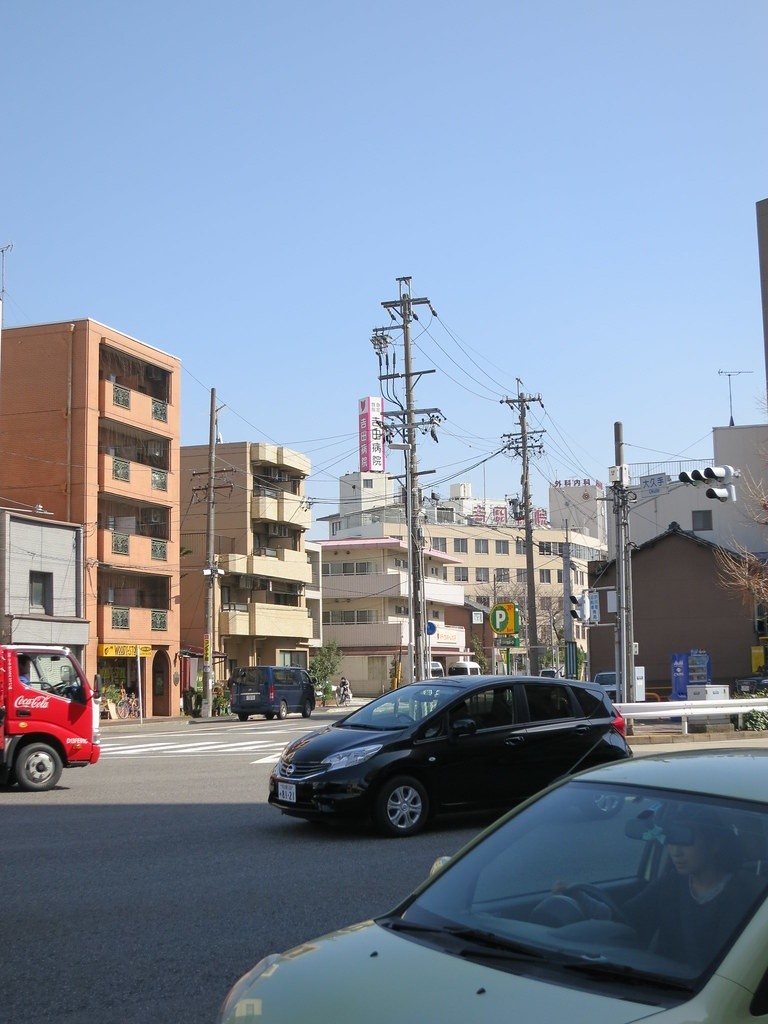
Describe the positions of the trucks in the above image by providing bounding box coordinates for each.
[0,644,103,792]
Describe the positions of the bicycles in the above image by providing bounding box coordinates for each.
[116,693,139,719]
[336,686,352,707]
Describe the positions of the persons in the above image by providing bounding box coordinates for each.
[18,655,31,685]
[340,677,352,704]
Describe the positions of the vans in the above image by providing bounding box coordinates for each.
[229,665,316,721]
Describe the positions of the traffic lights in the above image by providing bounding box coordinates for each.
[678,466,737,503]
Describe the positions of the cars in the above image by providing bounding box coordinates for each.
[266,673,634,837]
[218,741,768,1024]
[594,672,622,703]
